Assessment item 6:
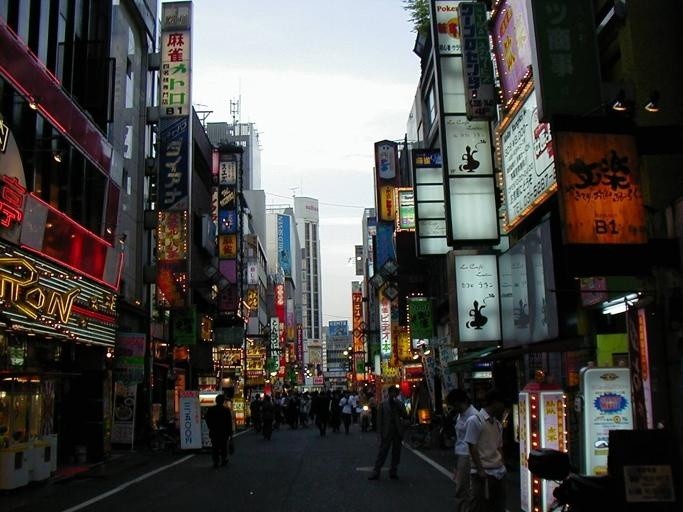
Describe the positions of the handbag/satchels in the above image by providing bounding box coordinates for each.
[229,439,235,454]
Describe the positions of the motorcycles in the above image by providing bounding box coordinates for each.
[527,440,608,511]
[355,403,373,430]
[407,411,455,450]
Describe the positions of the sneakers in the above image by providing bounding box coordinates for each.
[213,458,229,469]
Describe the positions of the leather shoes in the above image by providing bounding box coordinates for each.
[367,471,400,482]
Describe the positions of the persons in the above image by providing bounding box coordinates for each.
[250,389,376,440]
[366,385,407,481]
[445,387,479,512]
[204,393,232,469]
[463,387,508,512]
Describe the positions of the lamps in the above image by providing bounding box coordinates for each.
[50,148,68,163]
[635,89,665,114]
[113,233,127,245]
[15,92,46,110]
[602,293,639,315]
[98,222,116,234]
[607,89,631,113]
